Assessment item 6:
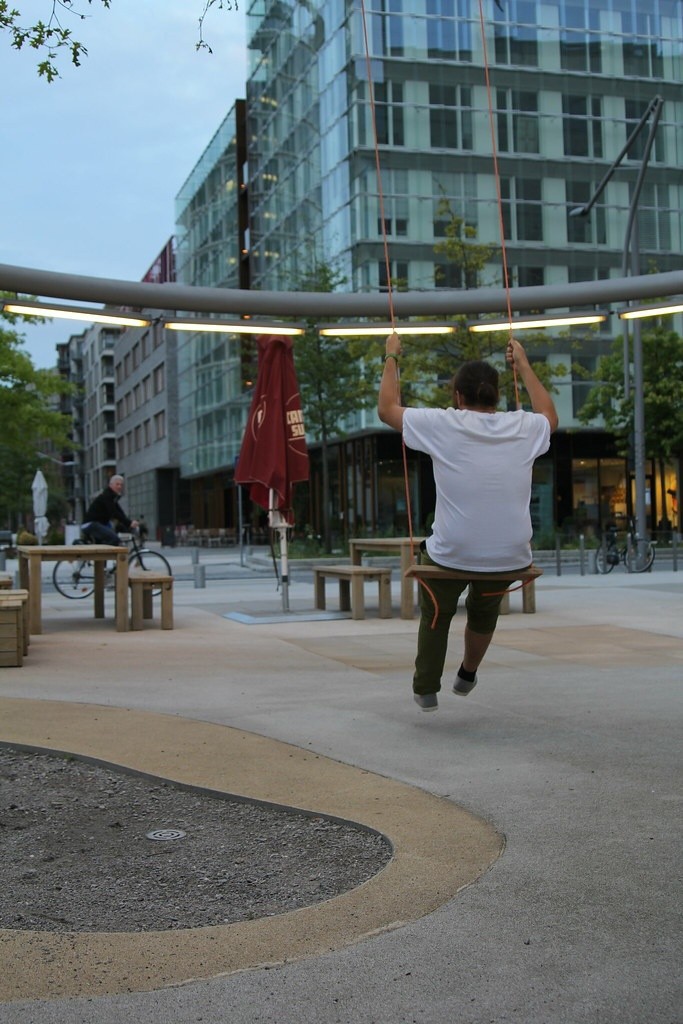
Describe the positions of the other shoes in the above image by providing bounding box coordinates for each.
[413,693,439,712]
[452,676,478,696]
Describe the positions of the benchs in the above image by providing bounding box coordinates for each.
[311,564,392,620]
[127,570,174,631]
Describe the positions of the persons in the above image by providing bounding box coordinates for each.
[139,515,149,546]
[377,332,559,712]
[80,475,139,587]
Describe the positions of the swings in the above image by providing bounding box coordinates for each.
[359,1,543,581]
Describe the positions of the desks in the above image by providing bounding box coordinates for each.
[15,545,130,636]
[346,537,429,621]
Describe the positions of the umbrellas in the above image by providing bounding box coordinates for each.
[32,471,50,545]
[234,335,310,612]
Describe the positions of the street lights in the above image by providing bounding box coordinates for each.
[568,91,665,528]
[16,451,81,536]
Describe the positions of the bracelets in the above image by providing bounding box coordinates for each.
[385,353,399,365]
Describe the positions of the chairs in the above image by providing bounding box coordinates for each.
[177,529,238,548]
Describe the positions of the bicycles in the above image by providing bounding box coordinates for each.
[51,517,172,600]
[593,514,656,576]
